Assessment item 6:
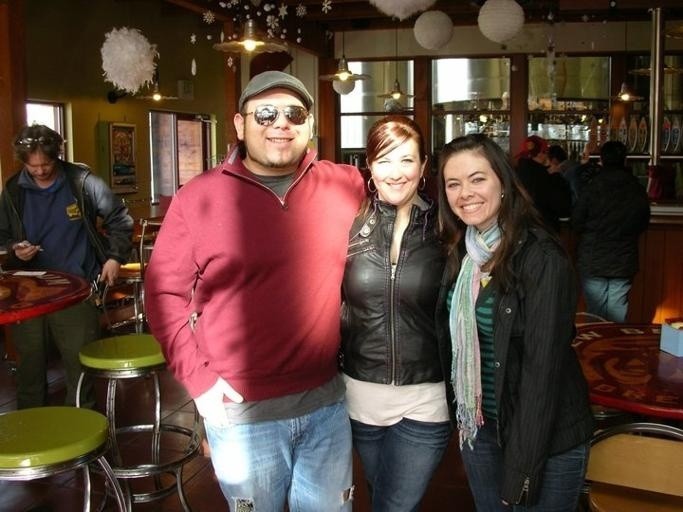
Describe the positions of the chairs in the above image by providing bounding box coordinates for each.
[576,418,682,510]
[96,213,165,339]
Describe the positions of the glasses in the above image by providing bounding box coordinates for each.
[239,104,310,125]
[14,135,58,152]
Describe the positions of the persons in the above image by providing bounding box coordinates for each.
[336,115,452,512]
[514,135,651,322]
[433,133,593,512]
[1,124,135,481]
[145,71,371,511]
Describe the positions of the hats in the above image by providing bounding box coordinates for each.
[517,135,546,157]
[237,71,314,112]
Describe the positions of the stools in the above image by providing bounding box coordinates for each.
[69,330,207,510]
[0,404,131,511]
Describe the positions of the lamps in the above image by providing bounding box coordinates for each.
[477,0,528,42]
[212,19,289,54]
[318,53,371,96]
[376,79,416,102]
[602,14,648,104]
[133,80,180,103]
[413,10,455,51]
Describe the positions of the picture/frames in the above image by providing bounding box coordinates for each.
[107,119,139,198]
